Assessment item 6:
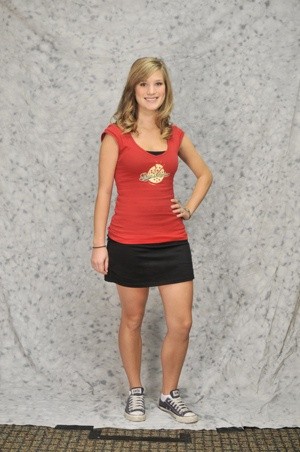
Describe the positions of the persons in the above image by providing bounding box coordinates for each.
[91,57,213,423]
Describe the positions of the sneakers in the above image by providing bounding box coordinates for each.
[123,387,146,421]
[159,389,199,423]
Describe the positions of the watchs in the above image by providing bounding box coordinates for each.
[184,207,191,220]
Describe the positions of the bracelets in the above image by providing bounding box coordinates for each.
[93,246,107,249]
